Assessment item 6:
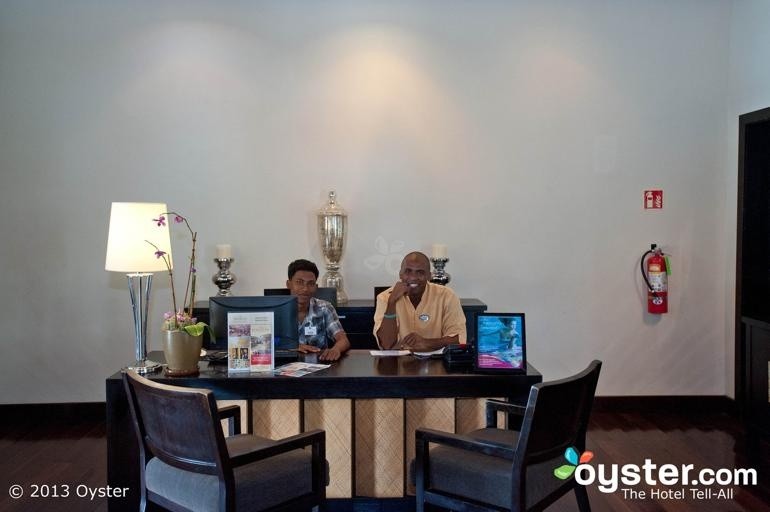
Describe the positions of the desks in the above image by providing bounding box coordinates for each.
[105,349,543,509]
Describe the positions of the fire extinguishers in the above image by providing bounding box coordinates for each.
[641,242,672,314]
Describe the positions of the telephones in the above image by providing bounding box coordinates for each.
[442,342,475,364]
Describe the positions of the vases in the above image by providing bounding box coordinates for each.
[161,327,204,377]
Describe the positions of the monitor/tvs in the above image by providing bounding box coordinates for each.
[209,294,300,349]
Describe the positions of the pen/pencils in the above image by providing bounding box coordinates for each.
[389,291,392,293]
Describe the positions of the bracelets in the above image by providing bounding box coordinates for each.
[383,313,399,320]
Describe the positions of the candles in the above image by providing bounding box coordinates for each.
[432,244,448,259]
[217,244,231,258]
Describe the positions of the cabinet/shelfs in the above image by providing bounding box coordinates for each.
[184,299,487,348]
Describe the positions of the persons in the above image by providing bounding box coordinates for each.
[370,249,468,353]
[285,258,351,361]
[482,317,521,350]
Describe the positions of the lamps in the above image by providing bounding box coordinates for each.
[105,201,173,376]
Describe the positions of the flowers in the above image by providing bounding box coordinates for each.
[144,211,217,345]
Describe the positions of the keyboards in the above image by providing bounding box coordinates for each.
[211,352,300,361]
